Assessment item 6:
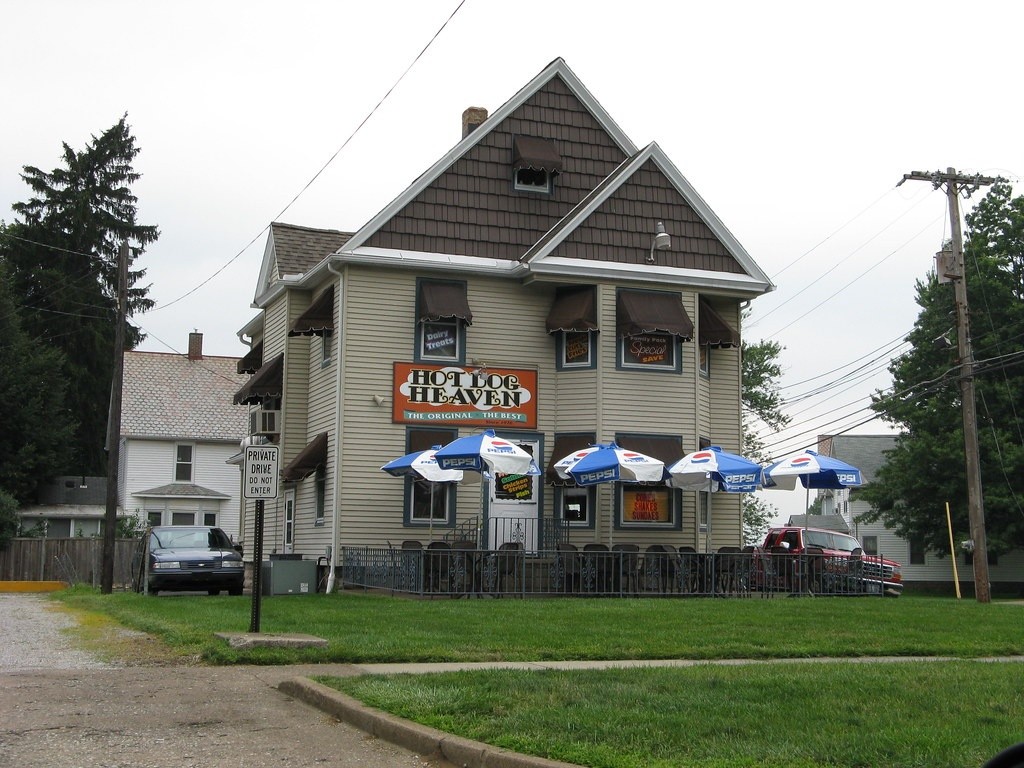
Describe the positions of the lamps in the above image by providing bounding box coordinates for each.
[374,395,384,406]
[470,358,487,379]
[646,221,671,264]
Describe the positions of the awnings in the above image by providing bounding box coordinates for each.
[419,282,473,327]
[280,433,328,483]
[545,285,600,336]
[699,294,741,349]
[233,353,284,405]
[237,340,263,375]
[617,290,695,343]
[546,438,685,487]
[287,284,334,337]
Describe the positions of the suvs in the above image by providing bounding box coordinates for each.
[754,528,904,599]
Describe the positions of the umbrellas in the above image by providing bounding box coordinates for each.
[554,441,673,552]
[762,450,869,554]
[665,445,763,554]
[380,445,464,543]
[434,428,541,549]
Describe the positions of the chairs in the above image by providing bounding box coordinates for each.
[553,543,754,597]
[755,545,863,599]
[387,539,520,599]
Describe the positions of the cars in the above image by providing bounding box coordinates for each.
[132,525,245,595]
